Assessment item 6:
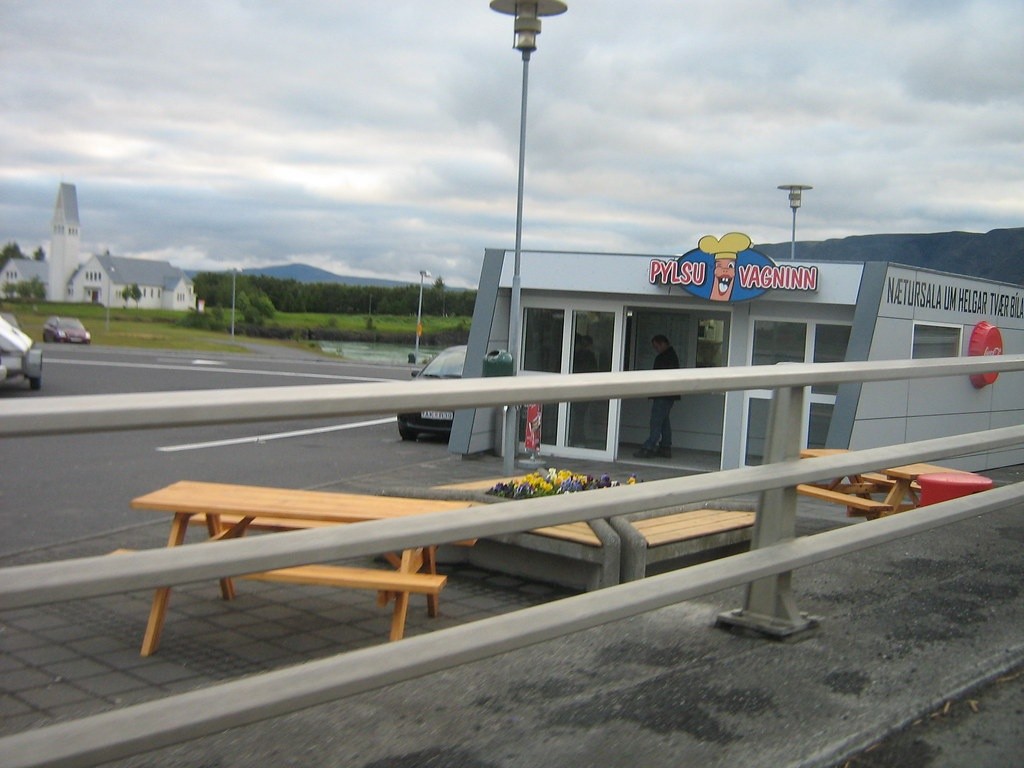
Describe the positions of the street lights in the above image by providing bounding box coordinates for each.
[230,266,242,338]
[488,0,568,472]
[413,268,432,366]
[777,183,814,259]
[106,266,115,330]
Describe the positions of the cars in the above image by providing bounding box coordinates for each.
[394,344,469,441]
[42,315,91,346]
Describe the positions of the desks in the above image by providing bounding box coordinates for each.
[139,478,487,658]
[799,447,978,522]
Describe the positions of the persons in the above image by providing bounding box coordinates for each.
[634,335,682,458]
[568,332,599,448]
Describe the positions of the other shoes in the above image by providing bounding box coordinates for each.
[632,447,652,457]
[655,448,671,458]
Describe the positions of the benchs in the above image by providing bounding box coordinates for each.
[610,500,759,581]
[381,468,551,500]
[797,472,922,512]
[103,513,480,595]
[433,517,620,594]
